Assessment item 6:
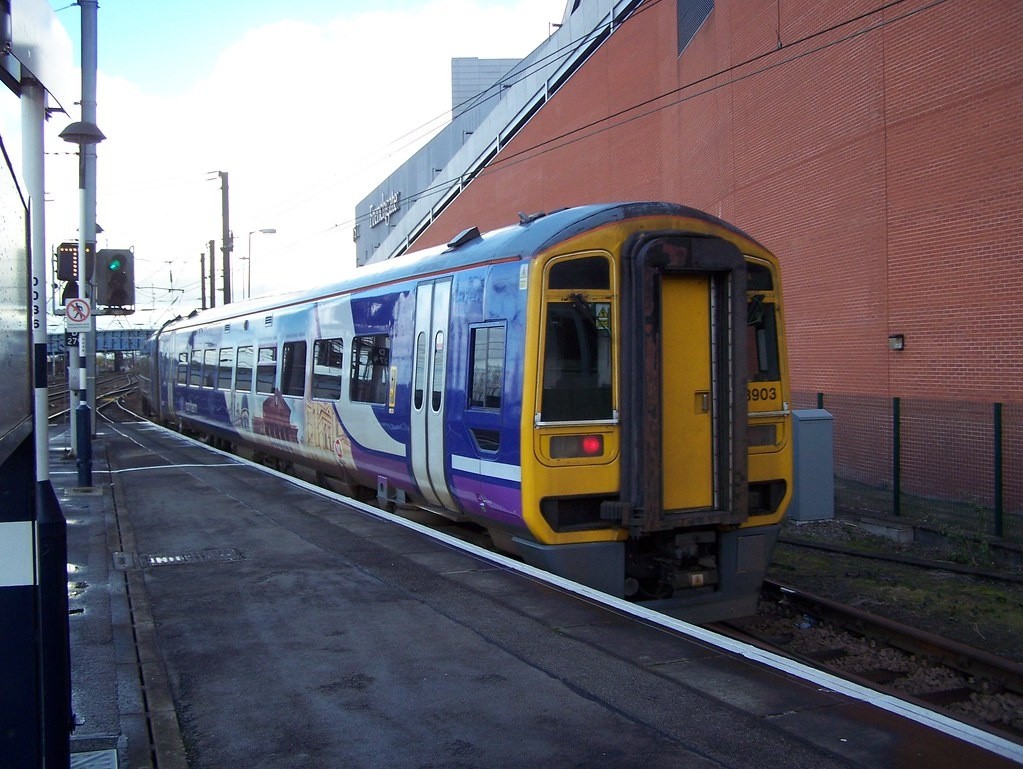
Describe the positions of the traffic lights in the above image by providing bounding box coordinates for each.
[56,242,96,305]
[96,248,135,307]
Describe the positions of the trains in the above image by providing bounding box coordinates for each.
[138,199,796,624]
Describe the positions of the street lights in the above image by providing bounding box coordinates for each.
[239,228,278,299]
